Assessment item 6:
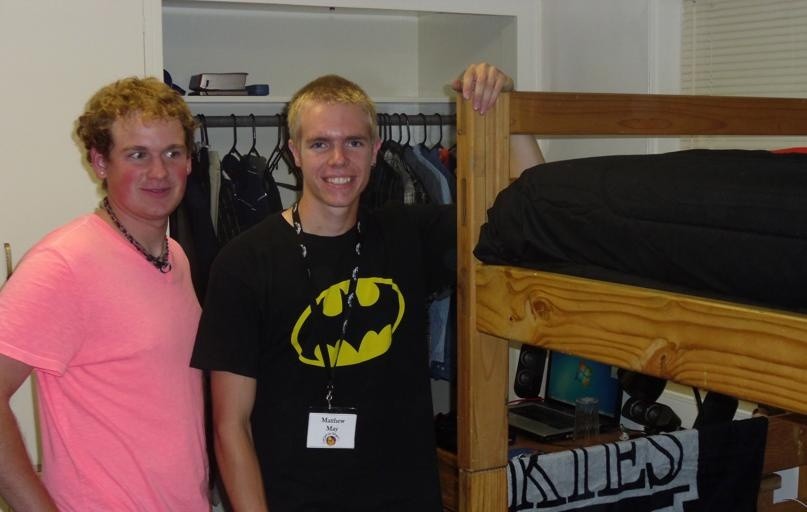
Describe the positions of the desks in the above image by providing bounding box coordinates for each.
[433,425,648,512]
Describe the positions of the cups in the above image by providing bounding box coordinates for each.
[573,398,600,446]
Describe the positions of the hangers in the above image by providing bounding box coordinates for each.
[199,111,457,191]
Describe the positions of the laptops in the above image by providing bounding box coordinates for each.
[507,349,623,442]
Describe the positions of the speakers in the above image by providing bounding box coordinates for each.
[622,396,681,430]
[514,343,547,398]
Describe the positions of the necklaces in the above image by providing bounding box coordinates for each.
[102,196,171,274]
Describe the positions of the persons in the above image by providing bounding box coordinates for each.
[186,57,548,511]
[0,76,213,511]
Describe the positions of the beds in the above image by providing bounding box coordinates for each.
[455,87,806,512]
